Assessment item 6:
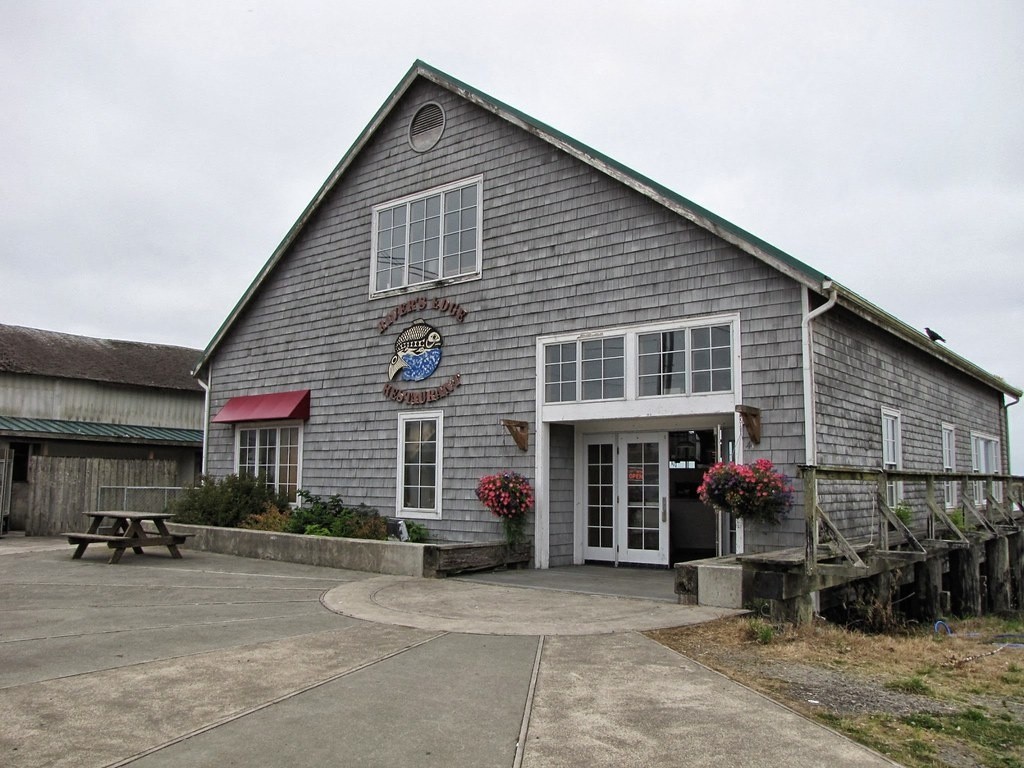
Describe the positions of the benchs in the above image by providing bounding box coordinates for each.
[145,529,196,545]
[59,532,132,550]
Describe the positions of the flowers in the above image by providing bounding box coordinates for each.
[697,457,796,528]
[474,470,534,542]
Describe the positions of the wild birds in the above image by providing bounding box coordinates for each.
[924,328,946,343]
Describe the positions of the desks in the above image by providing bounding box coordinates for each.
[73,510,183,565]
